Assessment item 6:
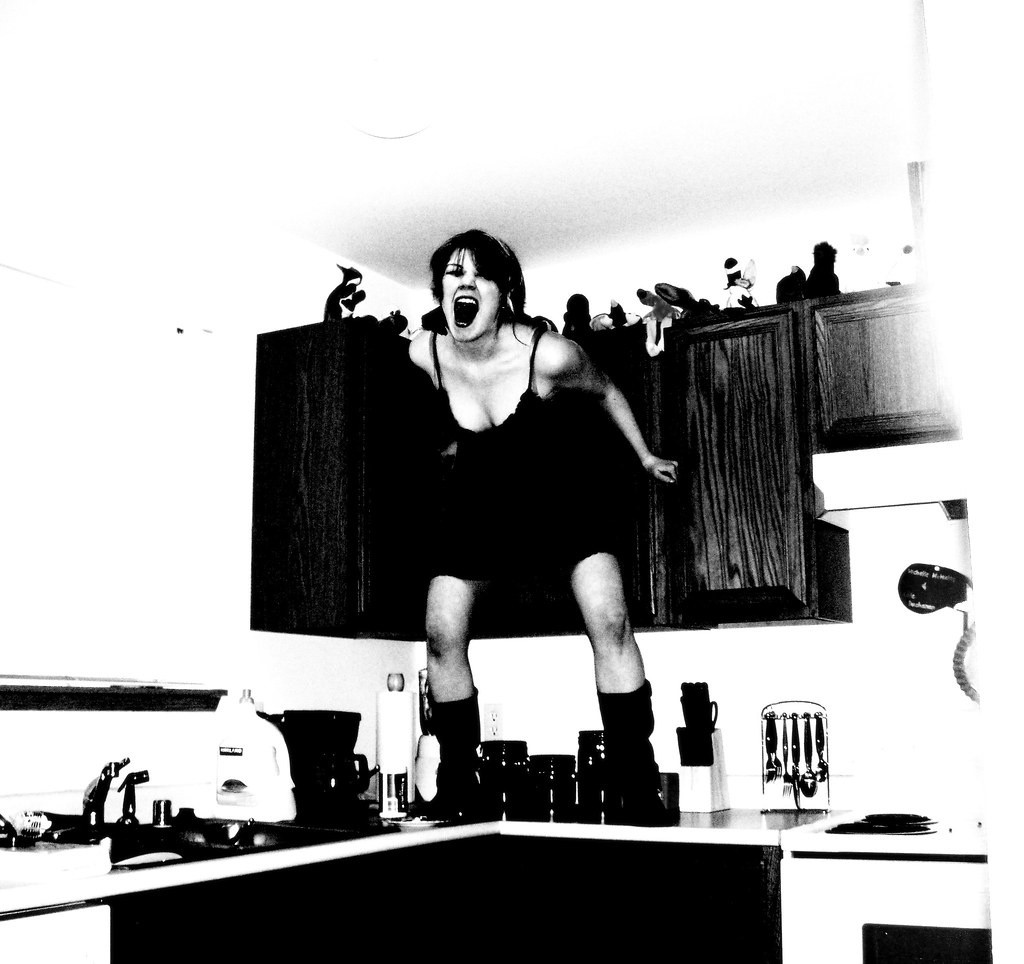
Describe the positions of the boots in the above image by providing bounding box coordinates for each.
[427,686,482,821]
[596,679,669,825]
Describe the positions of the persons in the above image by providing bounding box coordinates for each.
[408,227,678,827]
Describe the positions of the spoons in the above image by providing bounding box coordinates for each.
[814,712,828,783]
[800,712,817,798]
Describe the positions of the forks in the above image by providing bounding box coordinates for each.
[771,711,782,780]
[765,713,777,783]
[781,713,793,796]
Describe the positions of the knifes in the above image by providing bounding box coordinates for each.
[791,713,800,809]
[681,681,718,766]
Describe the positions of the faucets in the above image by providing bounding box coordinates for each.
[52,756,131,842]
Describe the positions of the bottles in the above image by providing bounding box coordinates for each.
[193,688,298,822]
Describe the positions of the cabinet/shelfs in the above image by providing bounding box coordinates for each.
[253,285,958,642]
[781,854,992,964]
[0,835,783,964]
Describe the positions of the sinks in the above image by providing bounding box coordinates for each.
[148,819,400,851]
[49,825,235,873]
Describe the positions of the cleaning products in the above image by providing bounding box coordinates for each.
[199,687,298,823]
